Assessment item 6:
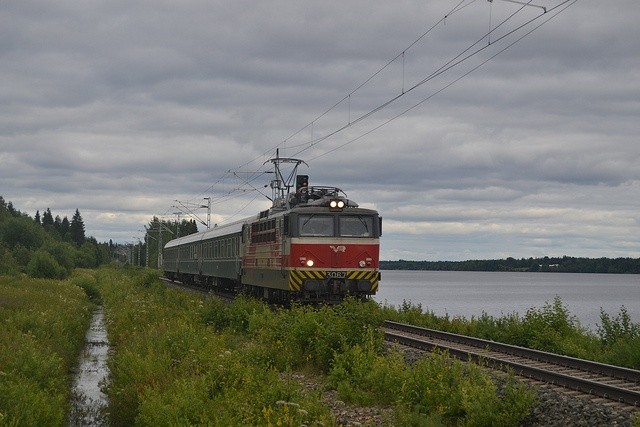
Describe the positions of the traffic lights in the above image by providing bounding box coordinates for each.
[296,175,308,192]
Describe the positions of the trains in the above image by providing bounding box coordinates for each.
[161,184,384,307]
